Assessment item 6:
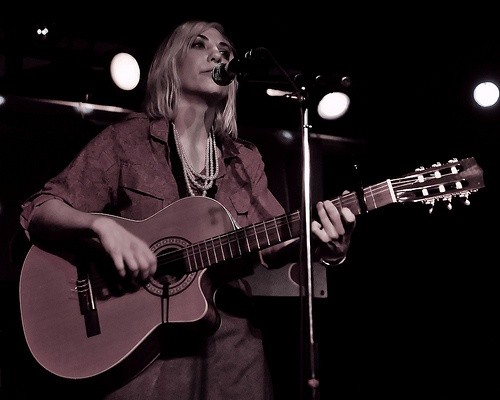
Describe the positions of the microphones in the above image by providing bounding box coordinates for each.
[211,45,263,86]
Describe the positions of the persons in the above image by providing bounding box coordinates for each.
[20,22,356,400]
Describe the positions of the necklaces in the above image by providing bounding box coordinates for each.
[172,122,219,197]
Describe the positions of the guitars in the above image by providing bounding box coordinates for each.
[18,155,487,392]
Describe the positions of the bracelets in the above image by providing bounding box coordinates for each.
[320,255,347,266]
